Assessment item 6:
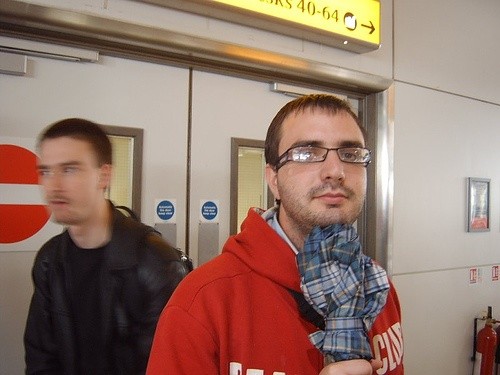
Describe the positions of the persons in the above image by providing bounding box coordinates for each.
[23,118,194,375]
[145,94,404,375]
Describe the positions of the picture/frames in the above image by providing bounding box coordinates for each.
[464,176,492,233]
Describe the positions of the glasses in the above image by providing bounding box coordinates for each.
[276,145,371,170]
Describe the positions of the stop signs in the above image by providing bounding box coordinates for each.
[0,143,53,244]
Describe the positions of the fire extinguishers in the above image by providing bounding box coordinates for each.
[473,316,500,375]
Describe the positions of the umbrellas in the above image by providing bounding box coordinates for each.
[296,222,391,375]
